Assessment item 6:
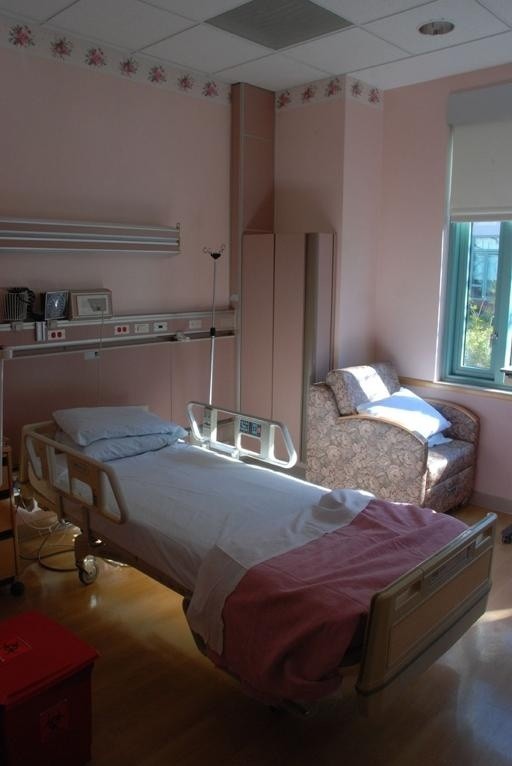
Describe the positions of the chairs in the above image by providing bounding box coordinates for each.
[304,362,482,515]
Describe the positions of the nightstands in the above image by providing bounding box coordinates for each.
[0,436,25,598]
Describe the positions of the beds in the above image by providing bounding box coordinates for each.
[19,418,496,728]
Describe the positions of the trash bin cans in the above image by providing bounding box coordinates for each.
[0,610,103,766]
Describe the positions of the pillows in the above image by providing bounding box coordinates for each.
[49,403,179,442]
[355,387,451,443]
[54,426,189,464]
[424,432,453,449]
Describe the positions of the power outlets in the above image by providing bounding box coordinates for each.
[84,349,102,362]
